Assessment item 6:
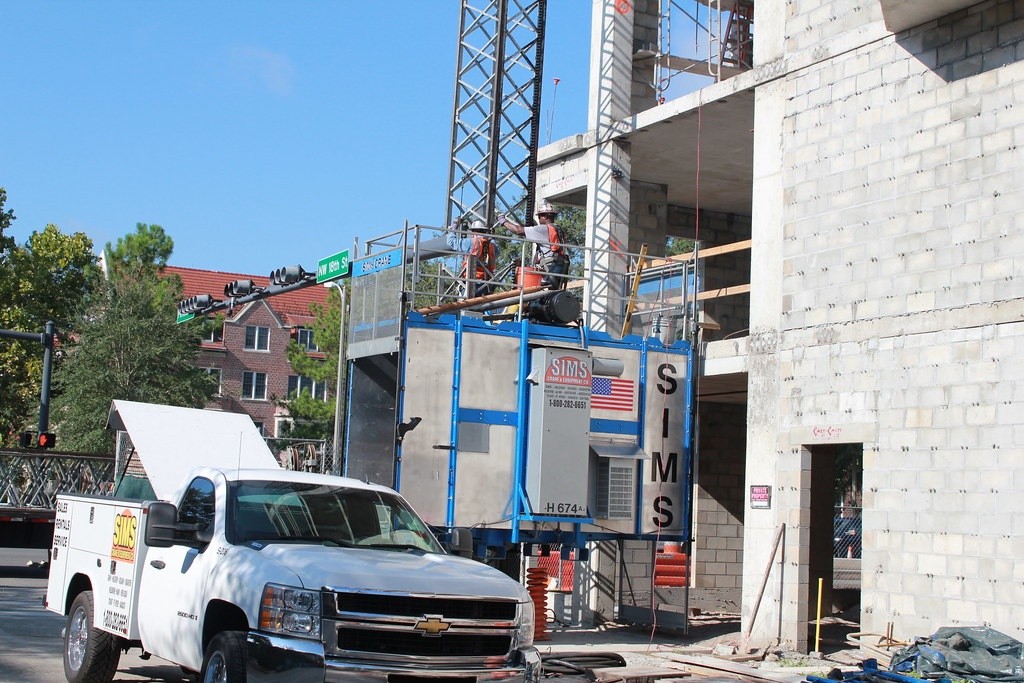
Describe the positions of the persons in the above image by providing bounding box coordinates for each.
[446,215,500,312]
[498,205,571,325]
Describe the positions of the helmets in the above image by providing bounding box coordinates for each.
[536,203,558,215]
[469,219,488,230]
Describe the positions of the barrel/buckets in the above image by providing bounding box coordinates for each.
[652,317,677,345]
[513,265,547,290]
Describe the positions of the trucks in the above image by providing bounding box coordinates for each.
[41,400,543,683]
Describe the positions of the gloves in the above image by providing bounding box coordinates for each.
[452,217,459,230]
[497,213,508,227]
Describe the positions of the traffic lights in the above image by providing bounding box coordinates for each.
[38,433,56,448]
[223,279,257,298]
[177,294,213,314]
[270,264,307,286]
